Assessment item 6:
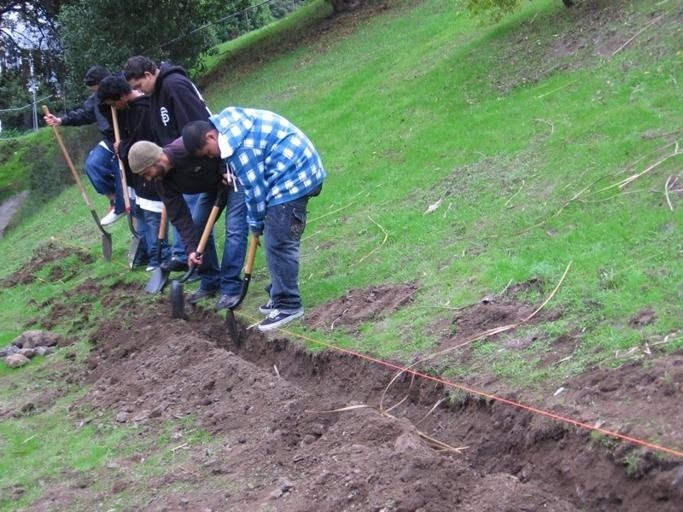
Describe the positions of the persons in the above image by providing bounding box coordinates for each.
[182,105,326,333]
[44,66,138,230]
[96,76,152,267]
[127,137,248,311]
[124,55,212,281]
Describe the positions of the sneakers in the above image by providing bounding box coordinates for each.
[161,256,187,271]
[258,299,275,314]
[184,270,200,284]
[99,205,125,226]
[186,287,216,304]
[145,266,160,273]
[213,294,241,311]
[257,308,304,331]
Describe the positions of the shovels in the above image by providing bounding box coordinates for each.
[167,191,226,321]
[108,104,143,270]
[143,203,168,297]
[224,229,260,350]
[41,104,115,265]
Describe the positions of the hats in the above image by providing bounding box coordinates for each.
[127,140,163,174]
